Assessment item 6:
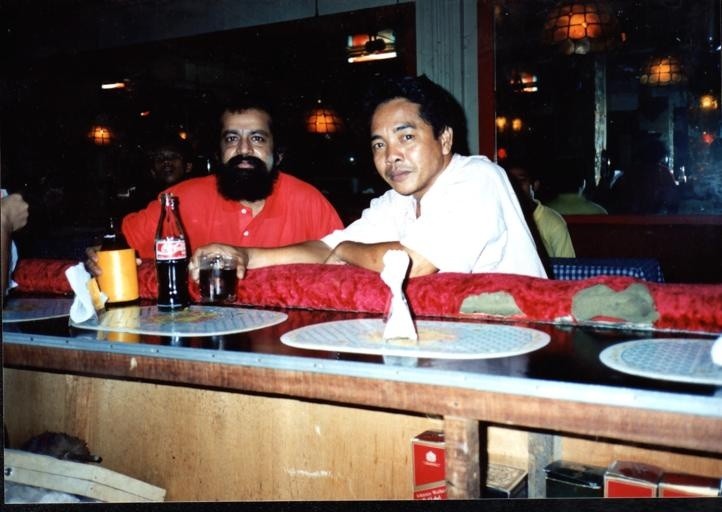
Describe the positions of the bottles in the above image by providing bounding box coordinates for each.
[96,215,140,305]
[153,190,188,313]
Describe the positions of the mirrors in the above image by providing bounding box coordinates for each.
[479,1,722,224]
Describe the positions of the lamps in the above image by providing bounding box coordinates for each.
[300,91,349,135]
[512,74,539,95]
[633,51,691,87]
[87,126,115,146]
[698,93,719,144]
[544,0,619,54]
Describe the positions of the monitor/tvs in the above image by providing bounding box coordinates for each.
[510,72,538,92]
[346,26,398,64]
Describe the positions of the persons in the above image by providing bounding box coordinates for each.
[1,187,32,312]
[85,90,346,280]
[546,155,607,218]
[498,153,576,259]
[188,73,549,284]
[103,134,195,234]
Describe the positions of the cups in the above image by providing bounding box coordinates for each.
[197,252,239,306]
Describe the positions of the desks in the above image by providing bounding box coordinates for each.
[547,258,662,285]
